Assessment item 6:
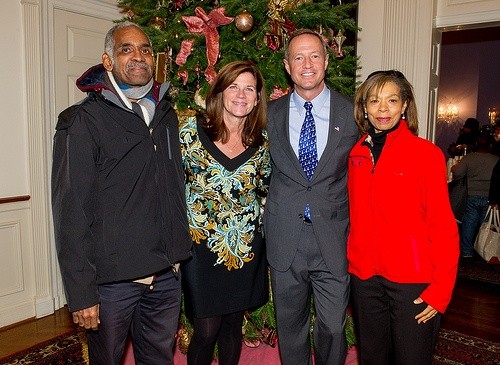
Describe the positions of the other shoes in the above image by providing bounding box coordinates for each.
[463,254,472,257]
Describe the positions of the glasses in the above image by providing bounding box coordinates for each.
[366,70,405,80]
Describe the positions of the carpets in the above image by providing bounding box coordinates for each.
[0,324,500,365]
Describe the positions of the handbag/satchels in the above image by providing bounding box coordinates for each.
[474,206,500,266]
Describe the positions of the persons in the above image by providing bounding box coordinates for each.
[51,21,193,365]
[447,118,500,258]
[178,60,272,365]
[347,69,460,365]
[263,31,359,365]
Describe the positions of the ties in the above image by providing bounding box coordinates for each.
[298,101,319,222]
